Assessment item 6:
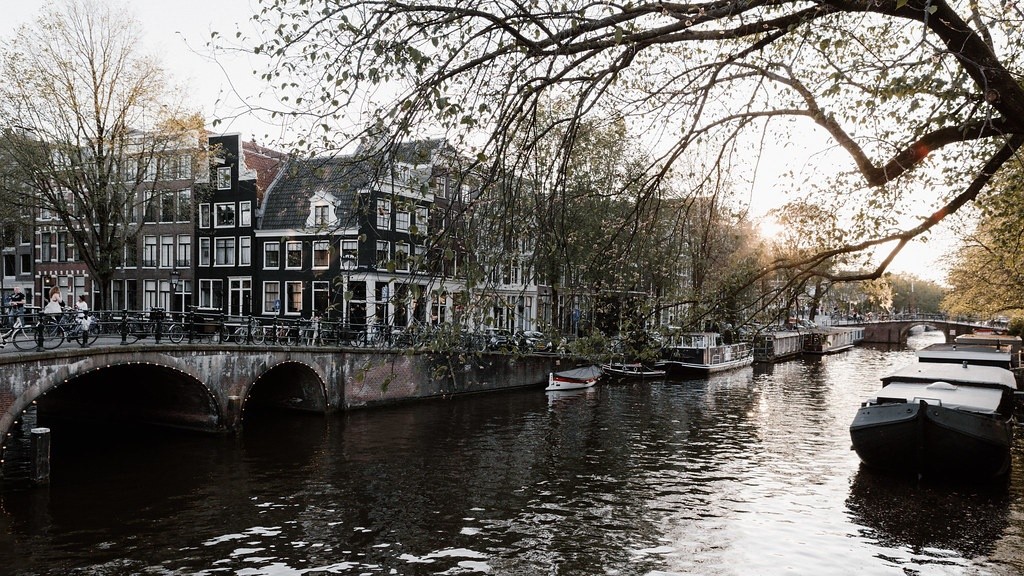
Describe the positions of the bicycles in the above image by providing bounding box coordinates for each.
[319,325,407,348]
[126,307,184,344]
[0,292,40,350]
[34,305,100,349]
[234,318,264,345]
[284,317,315,346]
[263,322,287,346]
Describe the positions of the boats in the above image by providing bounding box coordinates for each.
[602,363,666,378]
[800,326,855,353]
[743,331,805,363]
[850,362,1017,482]
[666,332,754,380]
[545,365,606,390]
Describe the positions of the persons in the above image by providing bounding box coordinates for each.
[848,310,889,323]
[303,314,326,347]
[43,286,63,316]
[9,288,26,332]
[81,310,91,348]
[73,294,88,320]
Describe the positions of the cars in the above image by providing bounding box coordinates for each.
[513,330,552,352]
[989,316,1009,327]
[483,328,519,352]
[785,318,818,330]
[849,313,861,320]
